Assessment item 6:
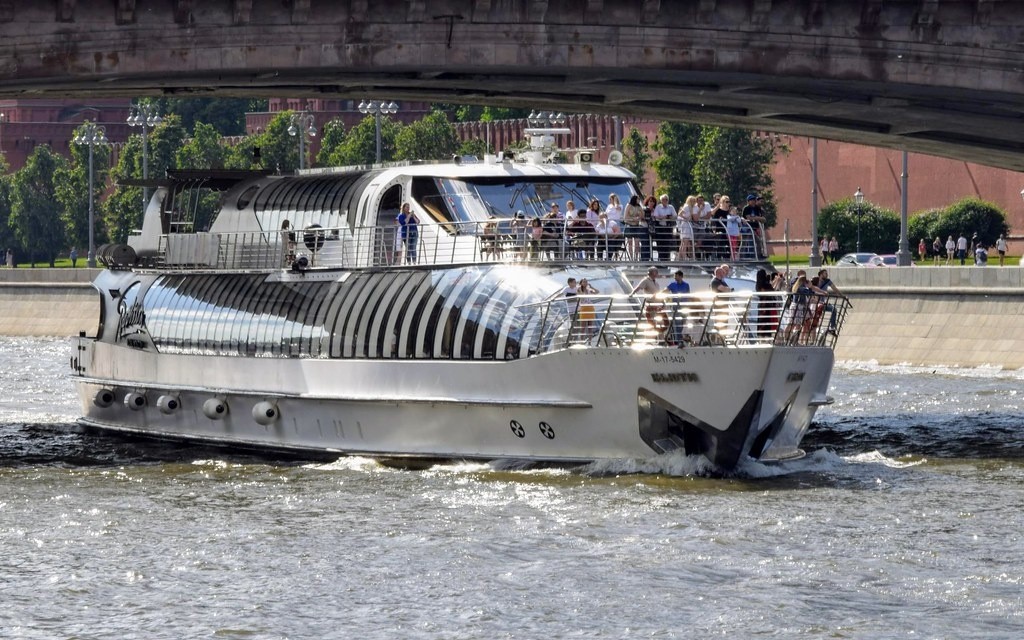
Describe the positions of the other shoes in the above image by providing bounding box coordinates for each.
[829,330,837,337]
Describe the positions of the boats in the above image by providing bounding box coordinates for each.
[63,127,852,465]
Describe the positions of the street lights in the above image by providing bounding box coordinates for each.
[288,111,318,170]
[356,98,400,164]
[74,123,108,269]
[125,102,165,217]
[853,185,864,252]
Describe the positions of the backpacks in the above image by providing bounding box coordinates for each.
[978,250,987,262]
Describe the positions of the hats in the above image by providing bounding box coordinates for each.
[747,195,755,201]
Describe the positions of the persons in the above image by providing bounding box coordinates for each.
[755,269,848,345]
[971,233,987,266]
[820,236,838,264]
[657,270,690,348]
[996,234,1009,266]
[552,278,599,347]
[630,267,660,345]
[399,204,420,265]
[70,248,77,268]
[710,265,731,340]
[510,192,770,261]
[280,220,293,268]
[919,239,926,262]
[946,236,954,265]
[955,234,967,265]
[933,237,942,265]
[484,216,502,259]
[6,249,13,267]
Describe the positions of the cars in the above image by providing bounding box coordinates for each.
[834,252,884,269]
[876,254,916,266]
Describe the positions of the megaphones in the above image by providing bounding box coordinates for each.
[453,155,462,164]
[578,152,593,163]
[609,150,622,165]
